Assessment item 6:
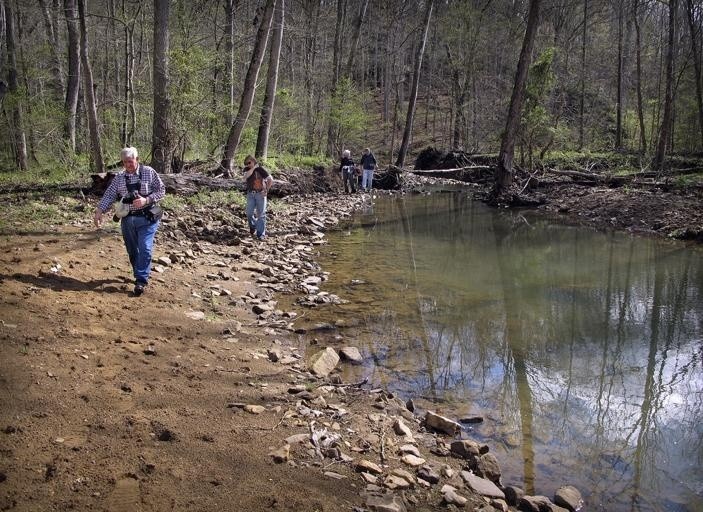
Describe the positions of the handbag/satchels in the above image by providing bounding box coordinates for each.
[148,206,162,223]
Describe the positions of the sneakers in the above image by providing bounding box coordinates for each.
[134,284,144,293]
[258,235,264,240]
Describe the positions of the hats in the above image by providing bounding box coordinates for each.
[115,197,129,219]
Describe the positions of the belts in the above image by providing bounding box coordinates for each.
[129,210,147,217]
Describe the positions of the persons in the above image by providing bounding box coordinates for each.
[337,148,357,194]
[356,148,378,189]
[240,154,274,241]
[92,145,168,294]
